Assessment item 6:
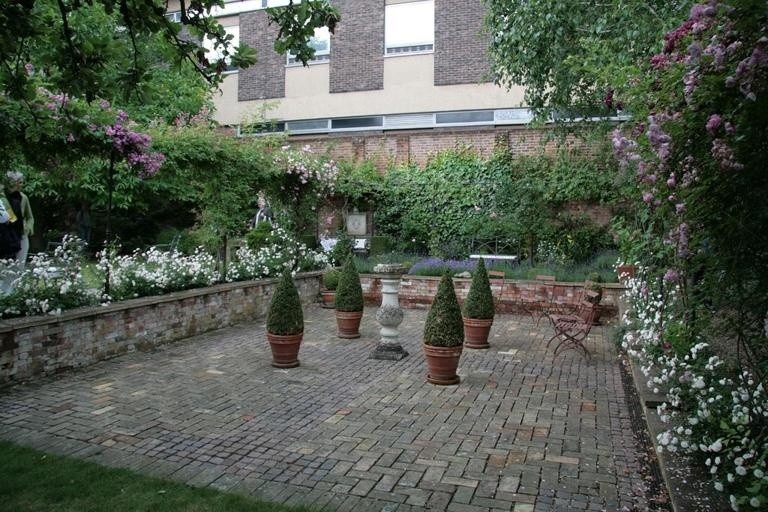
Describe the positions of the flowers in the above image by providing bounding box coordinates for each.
[372,262,403,273]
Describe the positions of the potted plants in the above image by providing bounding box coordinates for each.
[333,252,363,339]
[584,283,604,325]
[320,264,341,308]
[265,265,304,368]
[460,257,496,349]
[421,271,465,385]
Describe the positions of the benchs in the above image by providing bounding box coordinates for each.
[468,235,522,270]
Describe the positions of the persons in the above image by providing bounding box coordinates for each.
[249,198,271,231]
[0,168,36,267]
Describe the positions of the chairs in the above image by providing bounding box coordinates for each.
[488,271,600,364]
